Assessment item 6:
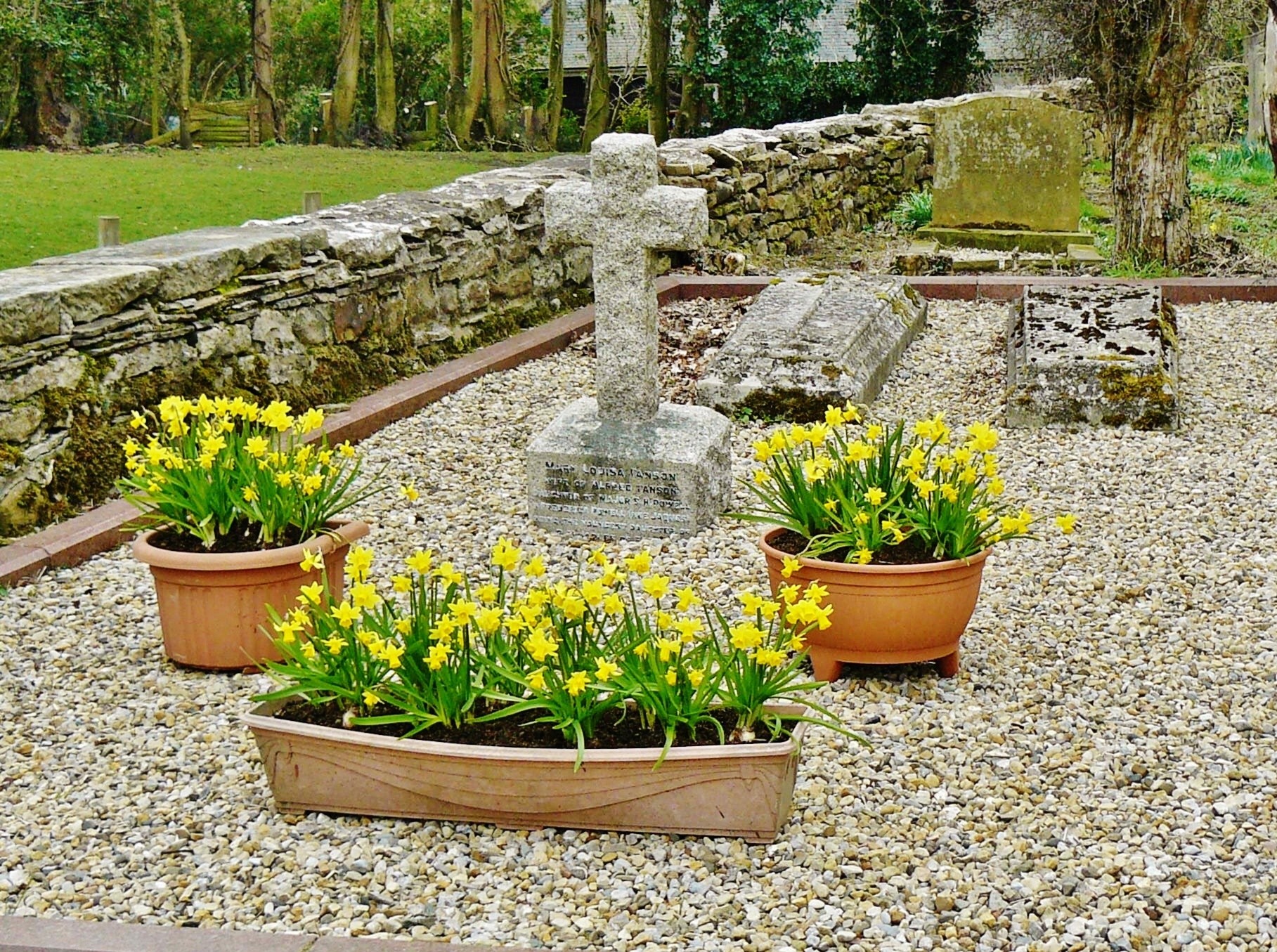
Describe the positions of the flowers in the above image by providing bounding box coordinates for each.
[236,532,875,762]
[714,398,1074,565]
[114,392,420,548]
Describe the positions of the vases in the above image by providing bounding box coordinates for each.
[759,527,994,682]
[241,705,808,844]
[132,518,371,666]
